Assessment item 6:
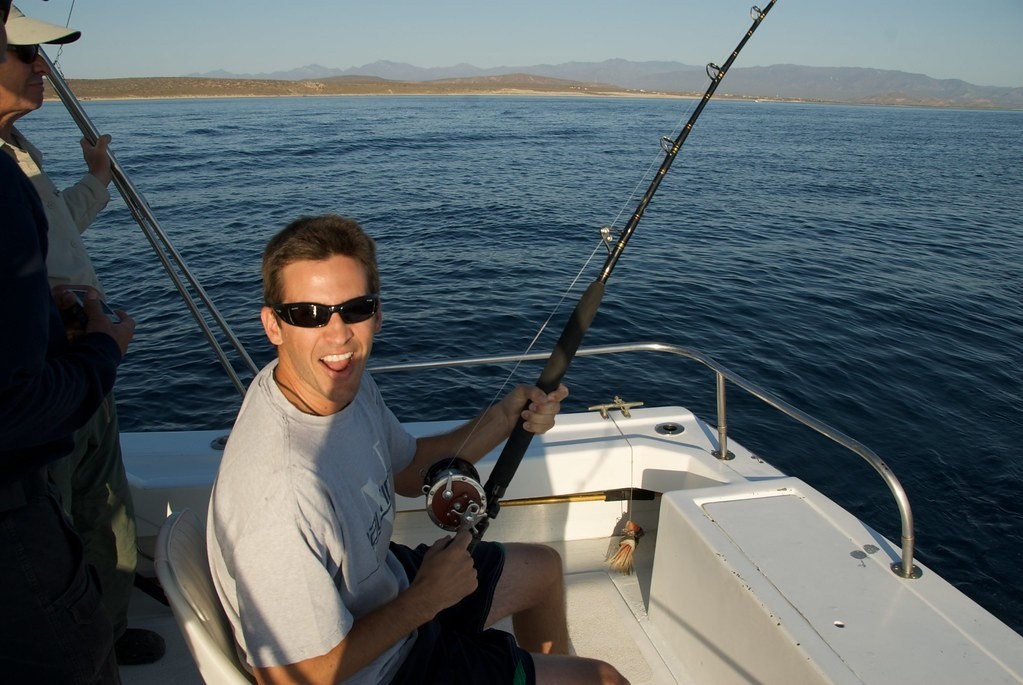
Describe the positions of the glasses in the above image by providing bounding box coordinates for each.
[7,44,40,64]
[266,294,378,328]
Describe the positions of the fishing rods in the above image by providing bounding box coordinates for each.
[420,0,778,557]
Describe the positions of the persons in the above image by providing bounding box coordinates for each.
[203,216,633,685]
[0,0,167,685]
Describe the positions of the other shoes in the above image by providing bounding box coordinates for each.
[115,628,166,665]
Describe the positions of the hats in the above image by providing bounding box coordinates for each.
[4,2,81,46]
